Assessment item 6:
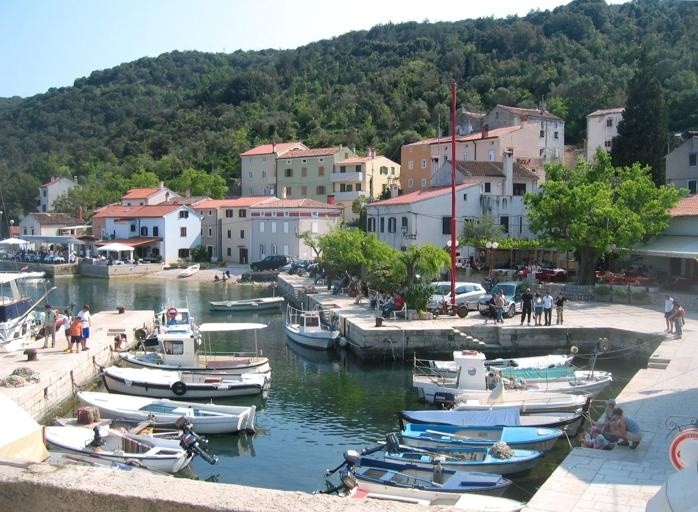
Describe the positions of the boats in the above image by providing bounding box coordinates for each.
[46,297,273,476]
[283,304,340,349]
[312,471,527,512]
[392,348,614,450]
[44,424,217,475]
[120,305,273,382]
[356,430,544,485]
[99,365,269,400]
[58,411,202,447]
[323,448,514,501]
[210,294,285,312]
[17,270,46,278]
[75,390,261,441]
[180,263,200,278]
[0,267,73,347]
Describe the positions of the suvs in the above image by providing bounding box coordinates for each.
[250,255,293,272]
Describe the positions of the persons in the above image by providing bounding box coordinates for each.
[663,295,686,340]
[353,280,368,304]
[42,305,59,348]
[484,254,568,326]
[68,317,83,354]
[215,270,231,282]
[369,290,406,319]
[615,263,657,281]
[60,307,73,353]
[456,255,486,271]
[0,246,115,265]
[114,332,128,349]
[77,304,92,352]
[313,271,326,286]
[287,257,315,276]
[575,399,644,450]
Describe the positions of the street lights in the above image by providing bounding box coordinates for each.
[485,242,498,281]
[445,239,459,280]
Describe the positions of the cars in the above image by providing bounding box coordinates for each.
[423,281,542,318]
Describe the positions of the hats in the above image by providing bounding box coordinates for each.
[607,399,615,405]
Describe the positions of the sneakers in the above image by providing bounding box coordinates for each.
[617,441,639,448]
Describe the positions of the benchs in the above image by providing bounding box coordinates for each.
[318,276,407,321]
